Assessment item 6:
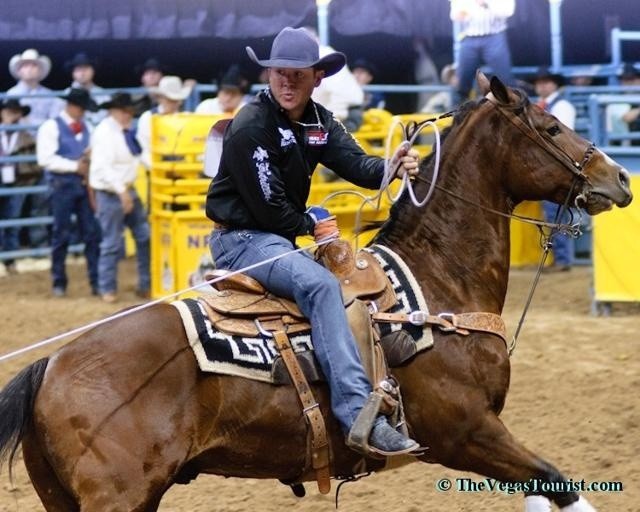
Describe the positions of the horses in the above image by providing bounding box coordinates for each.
[0,69,633,512]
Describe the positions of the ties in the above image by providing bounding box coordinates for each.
[72,121,82,134]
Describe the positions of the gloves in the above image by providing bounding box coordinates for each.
[305,207,330,223]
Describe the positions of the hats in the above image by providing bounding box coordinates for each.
[614,63,640,79]
[217,64,251,94]
[1,49,195,112]
[245,27,346,78]
[348,57,378,79]
[440,63,458,83]
[523,65,565,85]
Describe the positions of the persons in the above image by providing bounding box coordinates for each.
[0,0,640,307]
[202,24,422,457]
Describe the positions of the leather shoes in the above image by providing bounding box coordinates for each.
[368,416,420,453]
[542,266,569,273]
[100,290,117,304]
